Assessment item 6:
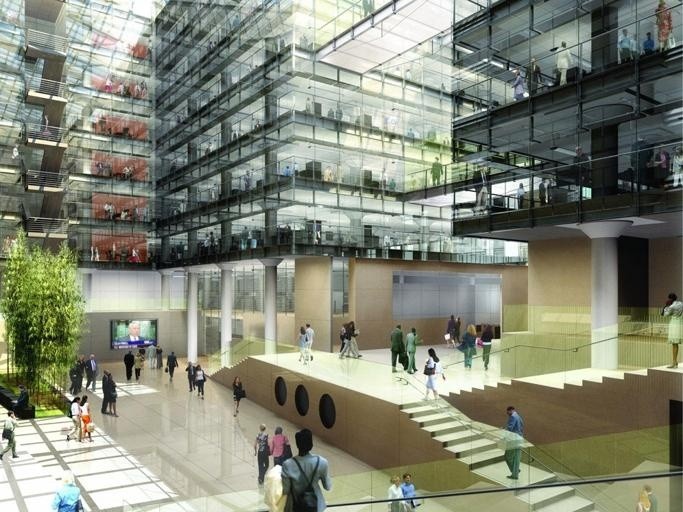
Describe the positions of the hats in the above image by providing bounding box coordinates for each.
[295,428,314,450]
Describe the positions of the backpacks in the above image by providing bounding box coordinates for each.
[290,455,320,512]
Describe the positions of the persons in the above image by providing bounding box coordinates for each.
[504,406,523,479]
[115,322,145,342]
[65,344,205,442]
[637,486,652,512]
[473,139,683,214]
[52,470,84,511]
[252,422,273,484]
[387,476,405,512]
[511,1,672,101]
[42,114,52,136]
[13,386,29,415]
[299,315,494,401]
[282,426,331,512]
[233,376,242,417]
[11,144,24,160]
[0,411,19,460]
[399,473,416,512]
[268,428,288,464]
[663,293,682,368]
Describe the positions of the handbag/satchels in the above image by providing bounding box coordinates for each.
[282,443,293,459]
[86,419,95,433]
[67,404,72,418]
[2,428,14,440]
[264,442,272,456]
[424,363,436,375]
[411,497,422,508]
